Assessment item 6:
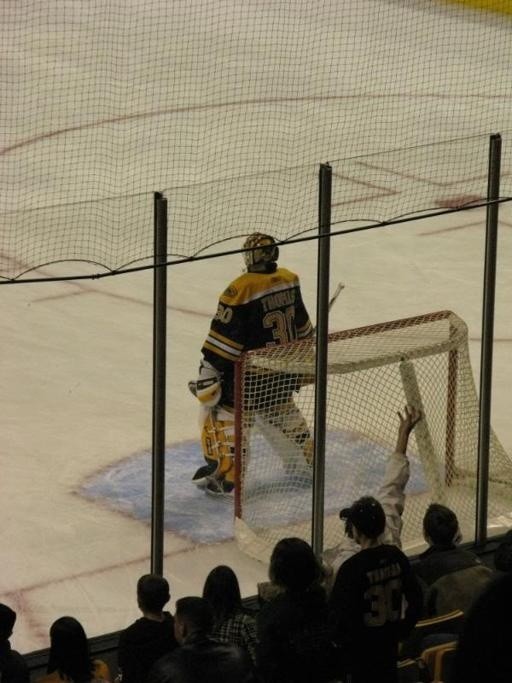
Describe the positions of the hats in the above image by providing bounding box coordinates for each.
[339,496,385,536]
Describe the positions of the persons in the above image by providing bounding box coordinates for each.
[188,233,314,497]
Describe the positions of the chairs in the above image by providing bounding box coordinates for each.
[396,607,465,683]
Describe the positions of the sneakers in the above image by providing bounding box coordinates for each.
[206,479,236,496]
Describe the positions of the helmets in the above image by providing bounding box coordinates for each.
[242,232,279,273]
